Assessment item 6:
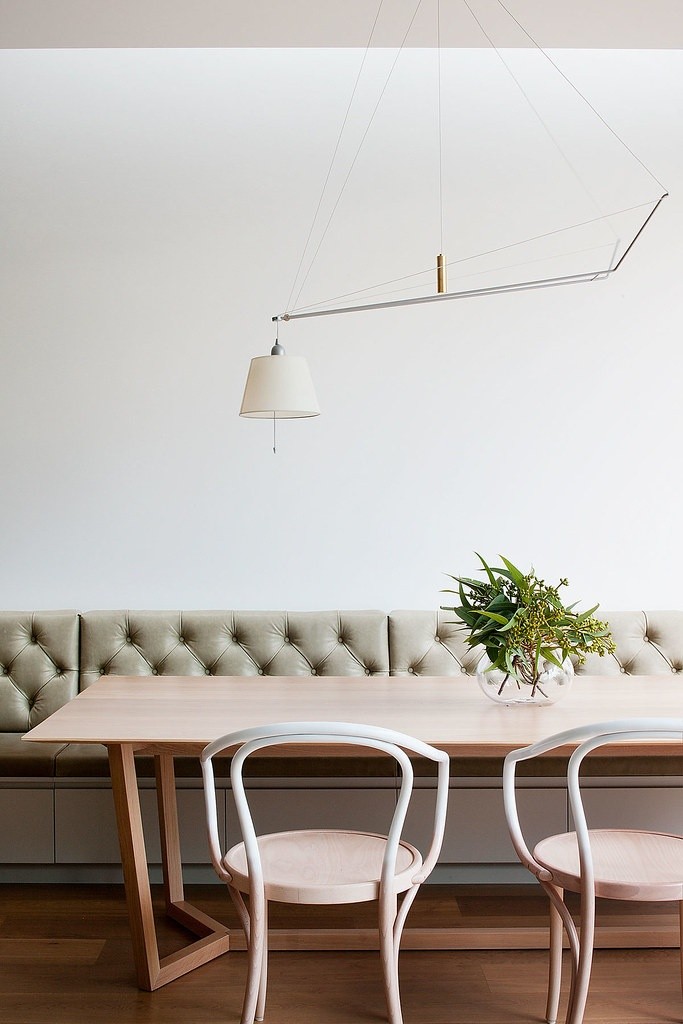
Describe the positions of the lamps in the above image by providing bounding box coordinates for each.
[240,0,669,455]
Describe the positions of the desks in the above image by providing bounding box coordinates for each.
[20,675,683,992]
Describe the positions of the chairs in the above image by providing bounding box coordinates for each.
[201,720,450,1024]
[502,719,683,1024]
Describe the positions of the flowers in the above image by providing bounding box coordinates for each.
[437,551,616,698]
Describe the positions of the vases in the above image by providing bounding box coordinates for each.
[476,642,575,706]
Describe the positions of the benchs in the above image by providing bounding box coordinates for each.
[0,608,683,886]
